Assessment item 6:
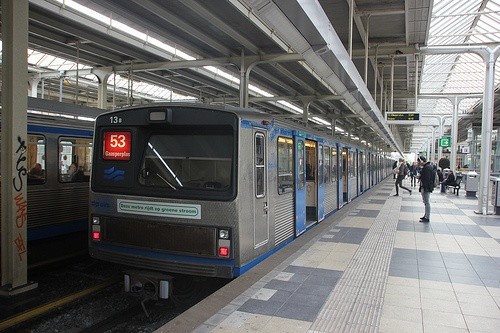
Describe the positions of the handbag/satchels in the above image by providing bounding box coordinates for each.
[404,165,409,175]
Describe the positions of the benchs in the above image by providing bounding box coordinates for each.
[446,175,464,196]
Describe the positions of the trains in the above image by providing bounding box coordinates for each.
[0,118,96,272]
[86,100,400,302]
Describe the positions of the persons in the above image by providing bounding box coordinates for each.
[62,163,81,182]
[440,170,455,193]
[393,158,411,196]
[392,157,442,192]
[29,163,44,181]
[306,164,314,180]
[417,156,433,223]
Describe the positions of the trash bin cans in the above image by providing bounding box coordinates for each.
[464,171,479,192]
[491,179,500,207]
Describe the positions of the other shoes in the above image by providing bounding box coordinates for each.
[420,217,425,220]
[419,219,429,223]
[393,194,398,196]
[409,190,411,195]
